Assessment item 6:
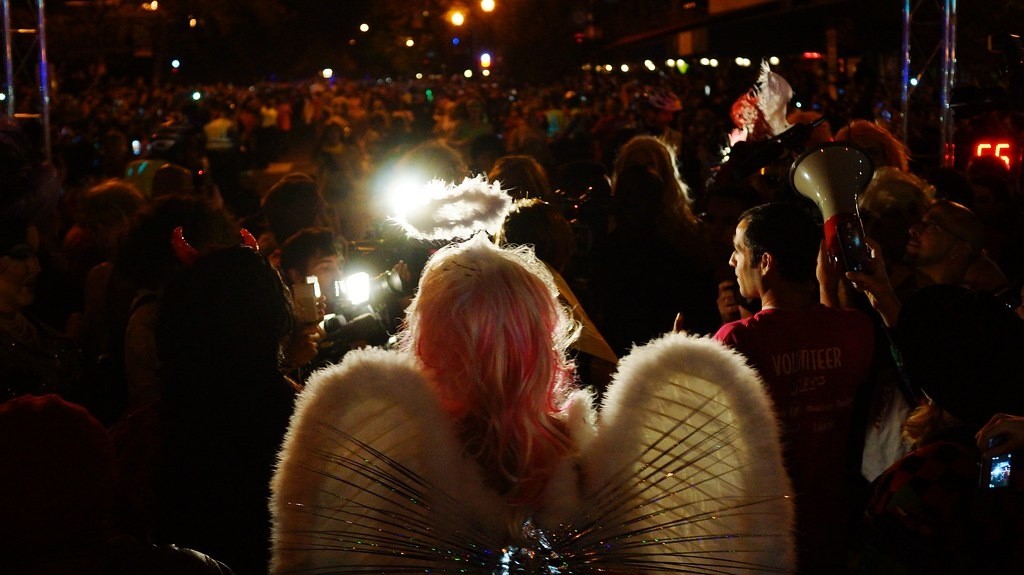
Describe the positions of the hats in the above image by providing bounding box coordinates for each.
[0,393,120,544]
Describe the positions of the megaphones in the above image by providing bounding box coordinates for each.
[789,142,874,274]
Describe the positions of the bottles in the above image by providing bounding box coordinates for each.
[759,55,769,90]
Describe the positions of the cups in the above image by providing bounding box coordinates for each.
[291,282,319,323]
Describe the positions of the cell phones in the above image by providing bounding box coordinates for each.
[980,432,1013,492]
[834,214,872,274]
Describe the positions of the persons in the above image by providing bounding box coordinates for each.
[0,69,1024,574]
[716,203,876,468]
